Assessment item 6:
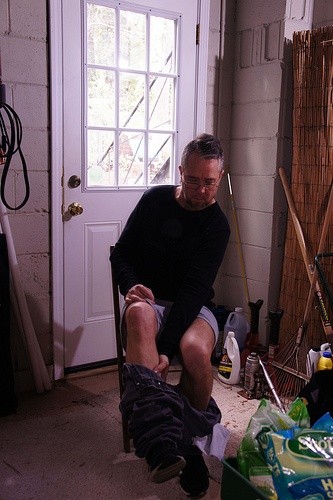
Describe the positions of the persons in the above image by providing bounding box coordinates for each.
[106,132,232,496]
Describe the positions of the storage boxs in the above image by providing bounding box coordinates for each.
[218,456,270,500]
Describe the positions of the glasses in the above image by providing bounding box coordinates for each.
[183,169,221,190]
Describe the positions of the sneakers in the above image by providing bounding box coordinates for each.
[178,444,210,496]
[150,453,187,483]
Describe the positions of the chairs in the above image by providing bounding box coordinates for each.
[106,245,215,455]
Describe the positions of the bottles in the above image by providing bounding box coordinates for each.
[304,346,333,404]
[244,352,260,395]
[223,307,249,353]
[210,305,232,366]
[218,331,242,385]
